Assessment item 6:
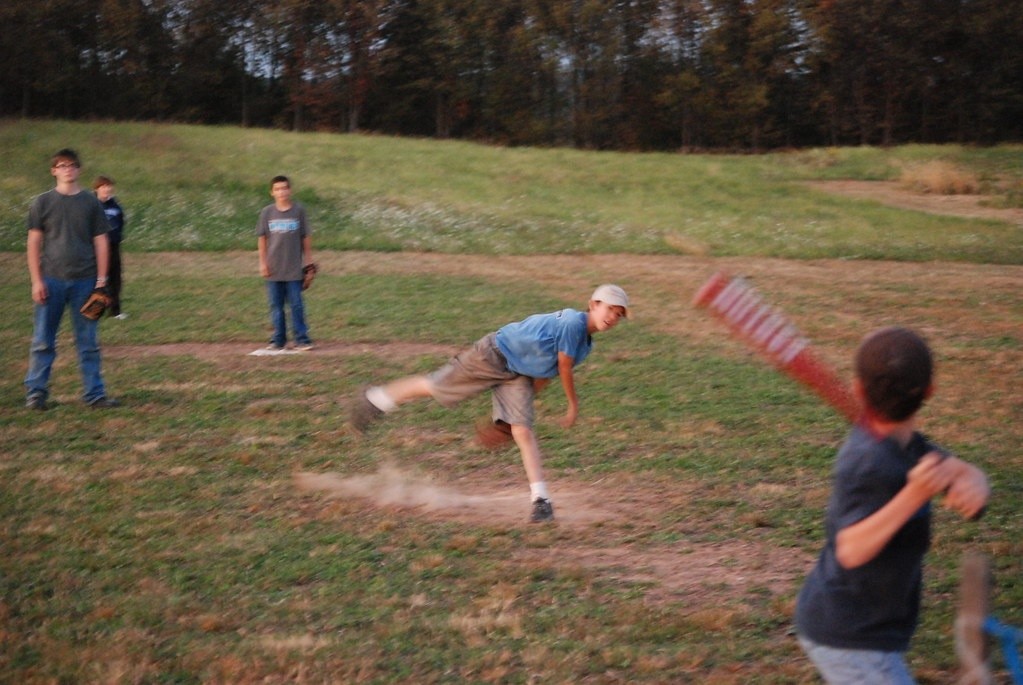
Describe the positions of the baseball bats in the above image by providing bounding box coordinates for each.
[690,266,990,524]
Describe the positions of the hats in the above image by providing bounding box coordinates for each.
[591,284,630,319]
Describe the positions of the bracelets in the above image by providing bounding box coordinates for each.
[96,277,107,282]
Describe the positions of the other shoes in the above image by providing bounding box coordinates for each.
[348,388,383,436]
[293,343,314,351]
[266,342,284,350]
[26,398,48,411]
[525,497,554,527]
[86,398,119,409]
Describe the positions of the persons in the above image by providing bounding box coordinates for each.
[94,176,129,319]
[784,329,992,685]
[254,175,317,350]
[23,148,116,410]
[353,282,630,523]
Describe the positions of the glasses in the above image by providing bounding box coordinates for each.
[55,163,78,170]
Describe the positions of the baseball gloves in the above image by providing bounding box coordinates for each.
[473,419,515,451]
[302,263,317,291]
[79,285,115,321]
